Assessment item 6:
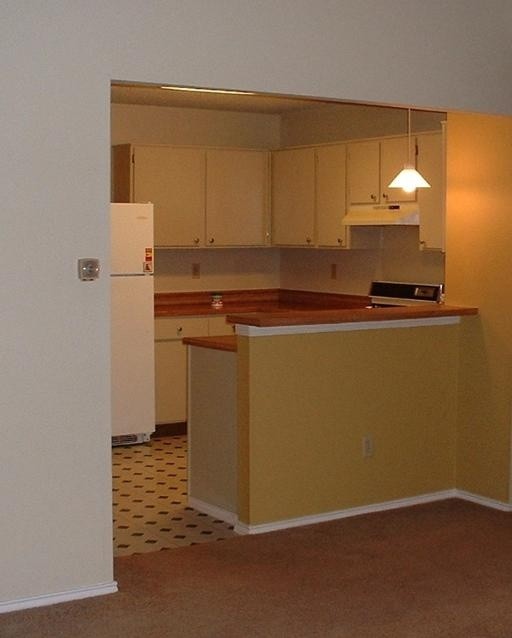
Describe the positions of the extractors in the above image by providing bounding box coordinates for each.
[339,201,419,228]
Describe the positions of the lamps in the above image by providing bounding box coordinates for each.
[387,107,430,193]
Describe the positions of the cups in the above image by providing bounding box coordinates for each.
[208,295,223,307]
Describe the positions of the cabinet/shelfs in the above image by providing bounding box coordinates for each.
[416,132,444,252]
[269,141,380,248]
[347,134,416,207]
[154,316,236,426]
[110,142,269,248]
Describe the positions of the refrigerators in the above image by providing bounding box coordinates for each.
[111,200,158,448]
